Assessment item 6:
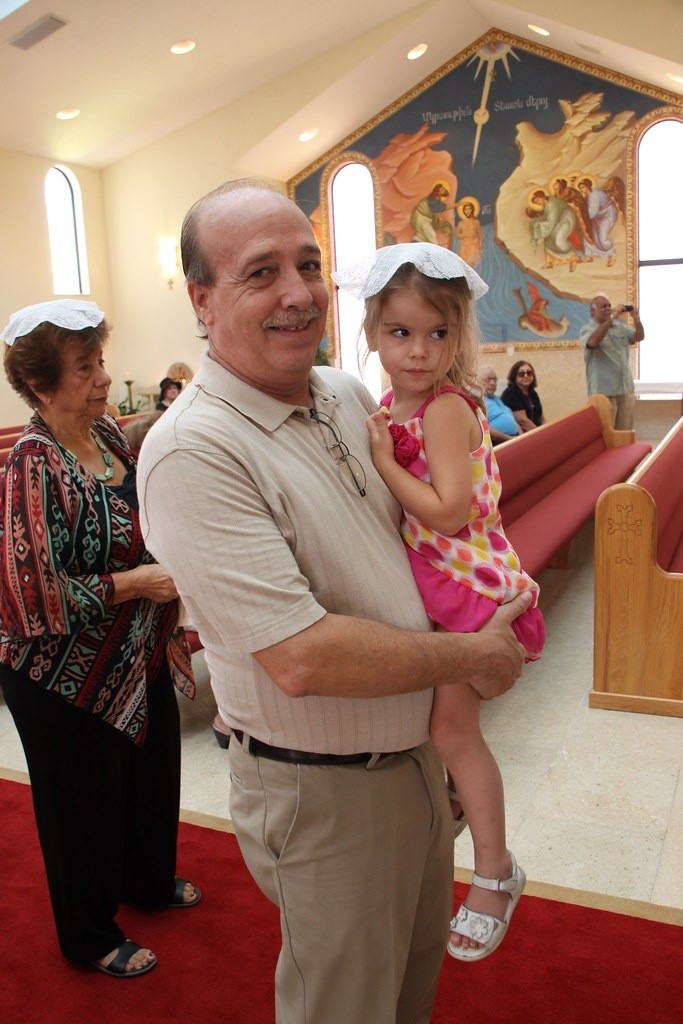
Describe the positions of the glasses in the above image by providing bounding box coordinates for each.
[518,370,535,378]
[480,377,497,382]
[309,406,367,498]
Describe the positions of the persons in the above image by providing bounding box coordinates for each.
[132,178,532,1024]
[155,377,182,411]
[579,294,645,431]
[330,243,546,961]
[0,298,204,978]
[467,365,523,446]
[500,360,547,432]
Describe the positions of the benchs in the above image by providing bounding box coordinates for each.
[589,414,682,718]
[0,412,154,473]
[492,394,653,578]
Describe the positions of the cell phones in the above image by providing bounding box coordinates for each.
[624,306,632,311]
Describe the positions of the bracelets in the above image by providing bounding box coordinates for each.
[610,313,613,320]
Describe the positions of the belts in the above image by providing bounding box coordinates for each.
[229,724,401,766]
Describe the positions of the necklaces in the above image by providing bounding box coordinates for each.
[89,428,114,481]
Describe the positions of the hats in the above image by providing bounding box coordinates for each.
[158,377,182,401]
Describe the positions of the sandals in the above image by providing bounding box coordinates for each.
[444,782,467,840]
[93,938,158,978]
[166,873,201,908]
[445,848,529,962]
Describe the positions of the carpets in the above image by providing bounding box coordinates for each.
[0,768,683,1024]
